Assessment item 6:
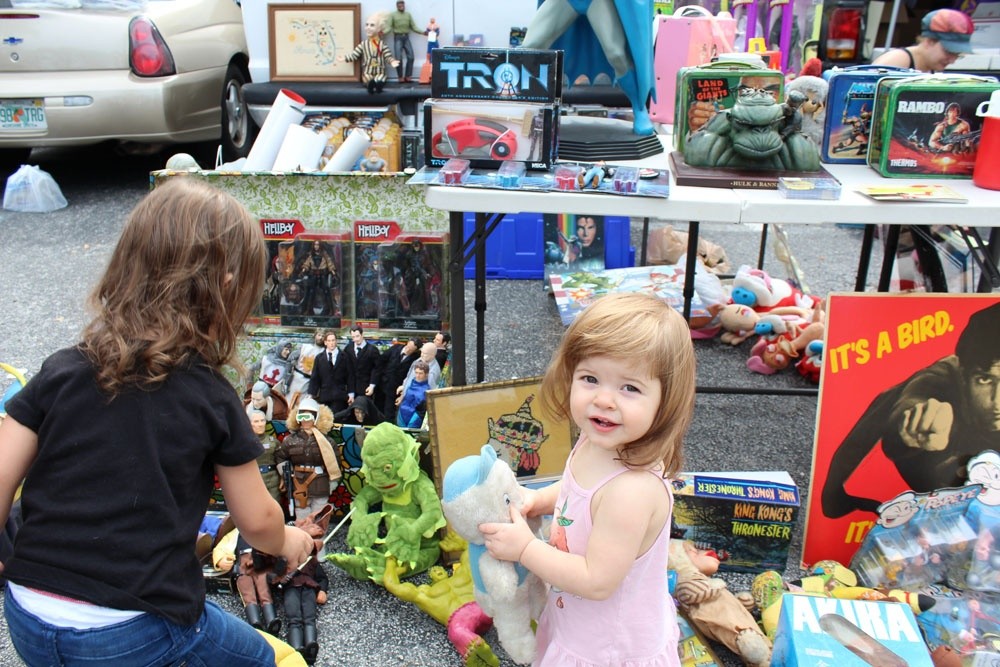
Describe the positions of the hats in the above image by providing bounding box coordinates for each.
[922,9,975,53]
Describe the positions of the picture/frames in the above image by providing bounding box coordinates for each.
[268,4,362,82]
[423,377,578,501]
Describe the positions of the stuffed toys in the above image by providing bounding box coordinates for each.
[441,443,551,665]
[783,57,831,156]
[665,537,773,667]
[721,264,827,383]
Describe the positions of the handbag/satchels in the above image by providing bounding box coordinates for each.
[3,164,69,212]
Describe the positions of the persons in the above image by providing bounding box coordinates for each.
[335,10,401,94]
[526,108,544,161]
[475,287,695,667]
[0,173,314,667]
[380,0,428,84]
[212,239,450,667]
[927,102,971,155]
[881,302,1000,488]
[578,160,615,188]
[869,6,976,75]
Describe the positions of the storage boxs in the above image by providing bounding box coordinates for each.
[423,46,565,167]
[669,470,799,577]
[463,212,635,281]
[151,170,461,525]
[769,592,935,667]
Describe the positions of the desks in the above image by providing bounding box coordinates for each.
[424,133,1000,389]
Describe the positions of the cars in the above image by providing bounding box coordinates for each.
[0,0,259,191]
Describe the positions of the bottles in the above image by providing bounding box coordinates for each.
[827,0,865,61]
[971,91,1000,190]
